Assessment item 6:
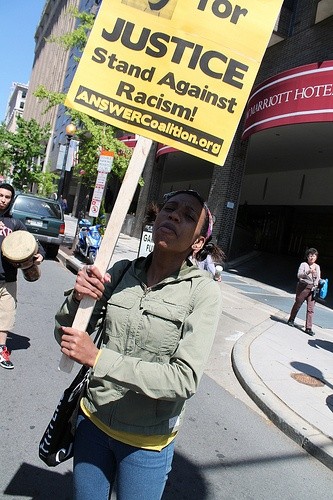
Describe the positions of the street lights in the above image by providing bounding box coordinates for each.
[58,121,76,198]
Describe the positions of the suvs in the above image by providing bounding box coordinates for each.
[8,193,65,259]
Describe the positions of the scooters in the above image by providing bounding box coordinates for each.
[75,218,104,264]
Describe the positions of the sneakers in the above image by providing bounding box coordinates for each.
[0,345,14,368]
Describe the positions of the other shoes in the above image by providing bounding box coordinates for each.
[305,328,315,336]
[288,318,294,326]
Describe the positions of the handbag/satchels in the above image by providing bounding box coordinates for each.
[39,376,91,467]
[319,279,328,299]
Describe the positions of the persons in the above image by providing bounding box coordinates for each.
[83,194,89,218]
[0,183,45,369]
[58,195,67,213]
[53,190,222,500]
[191,243,226,282]
[288,248,321,335]
